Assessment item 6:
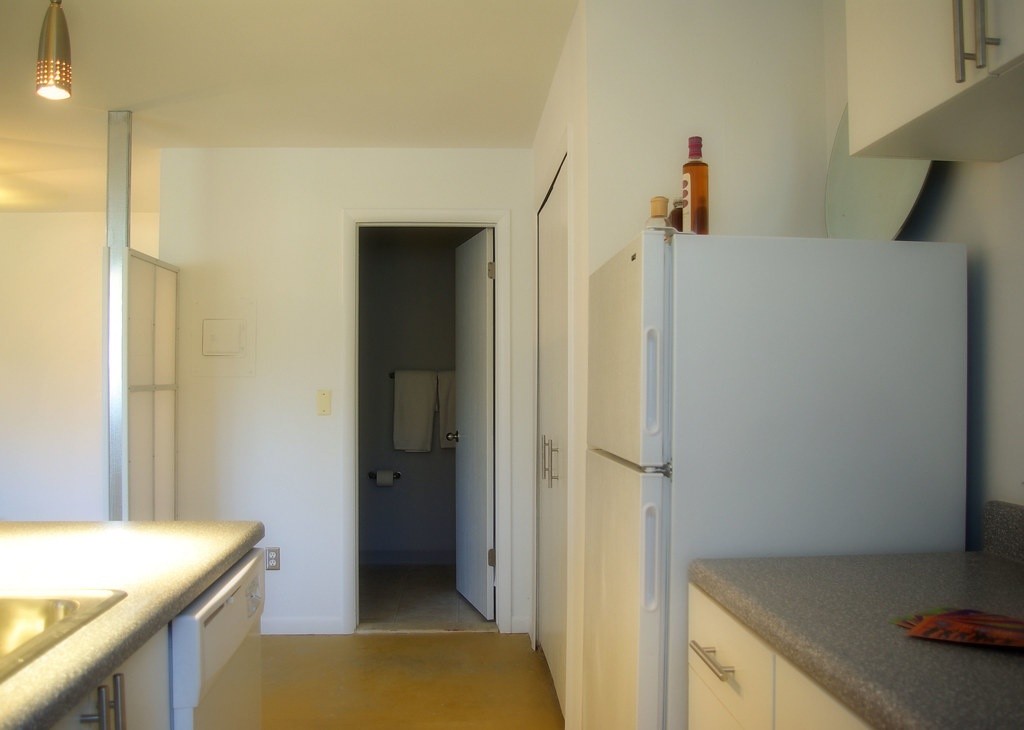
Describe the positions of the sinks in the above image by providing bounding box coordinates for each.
[1,589,127,687]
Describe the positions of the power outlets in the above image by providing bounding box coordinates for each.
[266,547,280,570]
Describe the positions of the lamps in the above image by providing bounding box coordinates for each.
[36,0,71,99]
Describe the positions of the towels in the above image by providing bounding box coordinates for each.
[393,368,437,452]
[439,371,458,448]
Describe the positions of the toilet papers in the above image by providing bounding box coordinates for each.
[377,469,394,487]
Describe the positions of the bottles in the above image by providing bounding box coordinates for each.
[667,199,683,233]
[681,136,709,235]
[643,196,673,228]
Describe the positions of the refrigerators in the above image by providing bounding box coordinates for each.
[581,232,967,730]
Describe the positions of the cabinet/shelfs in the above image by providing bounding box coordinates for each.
[48,623,171,730]
[843,0,1024,160]
[688,588,867,730]
